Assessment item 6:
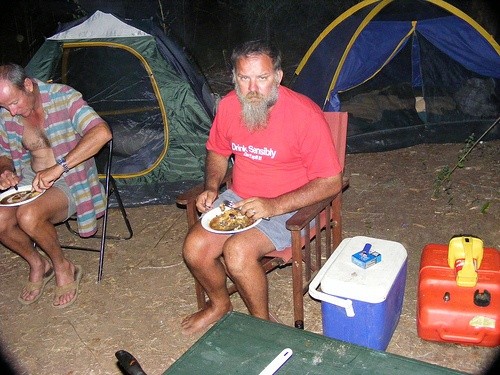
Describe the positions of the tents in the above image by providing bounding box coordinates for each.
[20,10,236,209]
[286,0,500,155]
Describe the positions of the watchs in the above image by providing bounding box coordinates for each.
[56,155,70,173]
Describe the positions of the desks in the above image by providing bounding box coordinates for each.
[164,311,465,375]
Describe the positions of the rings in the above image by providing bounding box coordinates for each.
[251,209,256,213]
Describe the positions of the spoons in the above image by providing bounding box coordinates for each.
[223,200,270,221]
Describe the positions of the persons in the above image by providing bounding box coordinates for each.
[180,38,343,335]
[0,62,113,309]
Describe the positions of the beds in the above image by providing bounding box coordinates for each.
[34,128,134,281]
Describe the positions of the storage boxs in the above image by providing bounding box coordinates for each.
[309,235,408,352]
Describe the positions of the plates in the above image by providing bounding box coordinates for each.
[200,206,263,233]
[0,185,46,207]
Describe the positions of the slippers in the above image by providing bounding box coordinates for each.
[52,264,82,308]
[18,269,55,304]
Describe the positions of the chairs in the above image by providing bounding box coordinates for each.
[176,113,349,330]
